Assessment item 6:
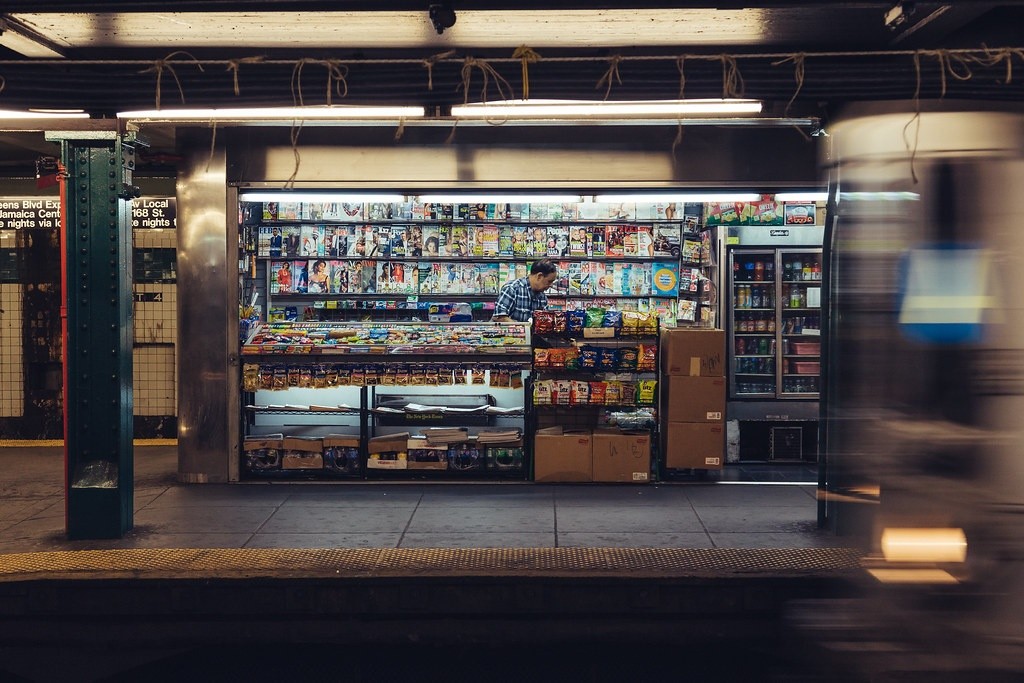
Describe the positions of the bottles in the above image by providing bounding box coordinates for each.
[487,447,524,471]
[735,337,768,355]
[782,284,807,309]
[734,284,775,309]
[782,255,821,281]
[734,256,774,281]
[783,377,818,392]
[325,446,358,472]
[734,311,775,333]
[735,358,775,373]
[782,317,820,334]
[246,449,276,472]
[736,383,776,393]
[448,444,479,471]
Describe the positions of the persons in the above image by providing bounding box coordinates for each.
[278,262,292,293]
[356,227,365,256]
[350,262,361,292]
[269,227,281,256]
[407,226,422,256]
[379,263,389,282]
[533,229,543,242]
[489,259,556,322]
[392,263,403,283]
[422,235,438,255]
[571,228,580,241]
[554,233,570,256]
[447,264,456,284]
[407,266,418,287]
[478,230,483,244]
[396,233,407,256]
[309,261,330,293]
[458,229,468,256]
[283,230,299,256]
[646,227,654,256]
[578,228,586,253]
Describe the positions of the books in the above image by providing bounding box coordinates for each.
[419,428,468,443]
[477,427,523,443]
[259,200,696,295]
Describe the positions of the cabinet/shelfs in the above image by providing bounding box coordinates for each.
[534,327,659,411]
[529,376,662,485]
[240,217,682,300]
[240,385,365,478]
[361,382,532,481]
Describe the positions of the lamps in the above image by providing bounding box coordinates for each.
[429,2,457,33]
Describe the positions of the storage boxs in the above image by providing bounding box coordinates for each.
[664,327,726,470]
[244,435,359,469]
[534,424,651,484]
[369,429,447,470]
